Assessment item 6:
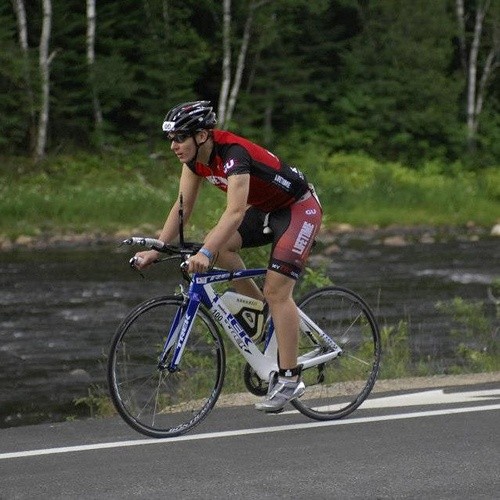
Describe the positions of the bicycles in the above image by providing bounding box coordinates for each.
[107,236,383,439]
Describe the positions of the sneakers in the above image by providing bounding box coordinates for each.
[256,373,307,412]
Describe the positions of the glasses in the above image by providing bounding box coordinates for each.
[165,132,192,146]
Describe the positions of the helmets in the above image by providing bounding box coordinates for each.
[161,100,218,131]
